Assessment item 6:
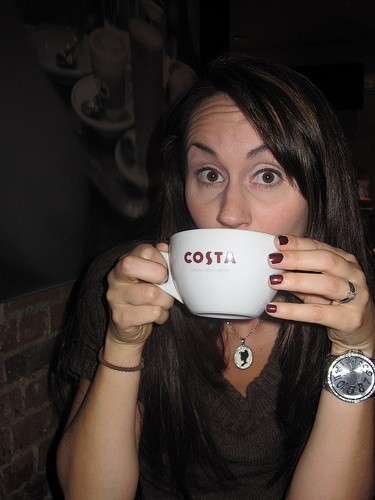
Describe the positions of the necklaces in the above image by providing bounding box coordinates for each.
[226,295,283,370]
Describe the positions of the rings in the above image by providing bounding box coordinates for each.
[339,280,356,304]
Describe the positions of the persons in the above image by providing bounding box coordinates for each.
[46,53,375,500]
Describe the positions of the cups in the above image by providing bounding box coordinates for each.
[151,228,283,320]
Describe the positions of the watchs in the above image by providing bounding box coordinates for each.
[321,348,375,403]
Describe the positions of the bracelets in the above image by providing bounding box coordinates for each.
[98,346,145,372]
